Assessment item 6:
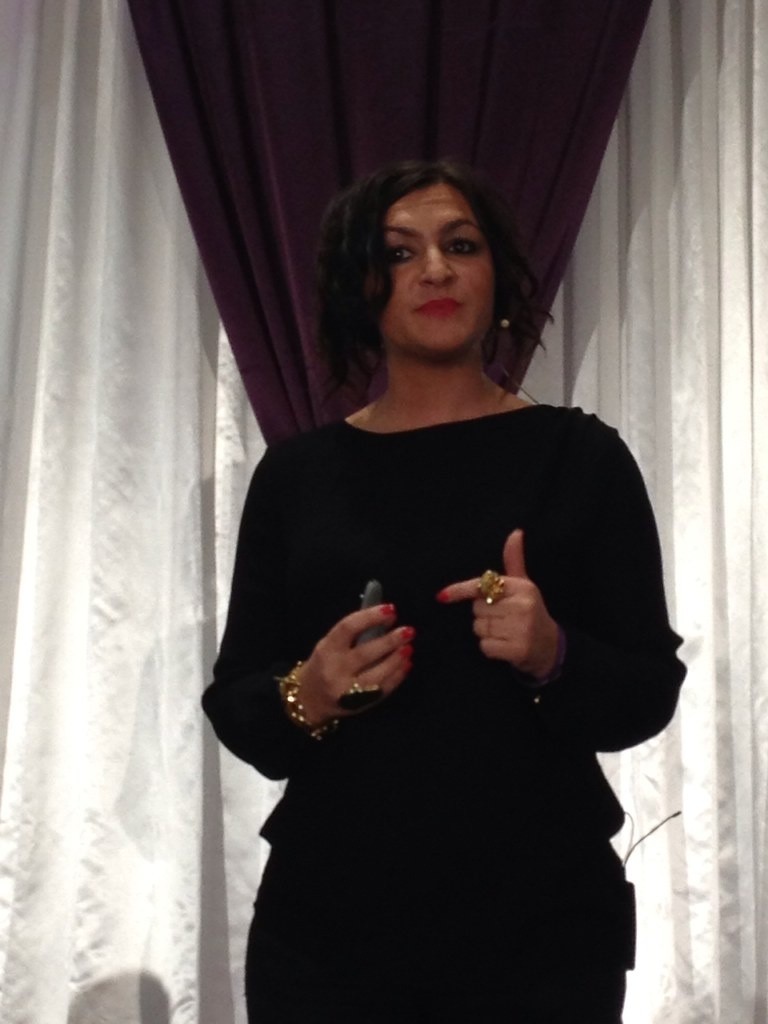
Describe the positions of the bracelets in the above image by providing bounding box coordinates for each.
[530,624,567,690]
[277,661,341,743]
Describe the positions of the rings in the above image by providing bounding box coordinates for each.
[474,568,505,604]
[337,681,384,716]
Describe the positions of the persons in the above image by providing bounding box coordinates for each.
[200,147,689,1022]
